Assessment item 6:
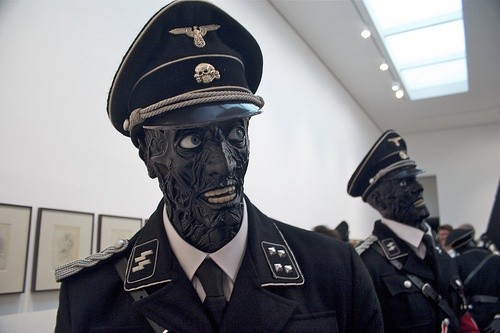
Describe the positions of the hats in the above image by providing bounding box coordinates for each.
[347,128,426,204]
[443,227,474,250]
[107,1,265,147]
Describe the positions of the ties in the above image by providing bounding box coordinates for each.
[194,258,227,333]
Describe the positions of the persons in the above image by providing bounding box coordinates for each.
[52,1,385,333]
[314,221,500,333]
[346,129,480,333]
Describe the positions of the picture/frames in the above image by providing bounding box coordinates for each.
[0,203,33,295]
[98,215,142,253]
[33,208,95,292]
